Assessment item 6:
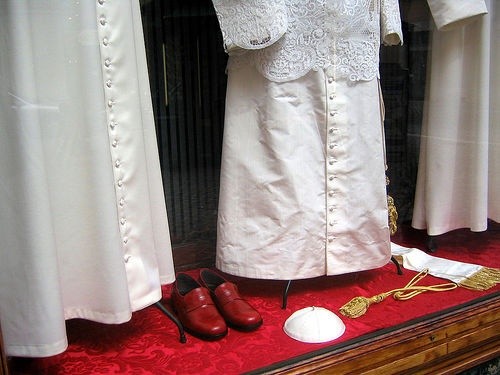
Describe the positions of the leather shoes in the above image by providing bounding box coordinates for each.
[173,272,228,340]
[197,267,264,331]
